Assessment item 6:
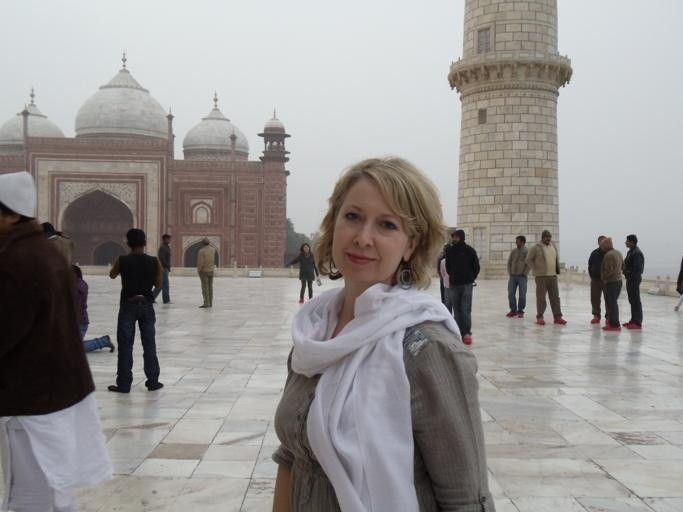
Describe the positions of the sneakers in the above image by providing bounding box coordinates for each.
[109,385,119,391]
[506,311,567,324]
[463,336,473,344]
[591,318,642,331]
[149,383,162,390]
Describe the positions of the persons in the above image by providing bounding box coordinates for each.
[505,235,529,319]
[284,243,319,304]
[273,158,497,512]
[673,257,683,310]
[105,228,163,392]
[68,264,115,354]
[1,171,116,512]
[153,234,176,305]
[587,235,646,331]
[436,229,480,344]
[40,222,73,267]
[524,230,568,325]
[196,237,217,309]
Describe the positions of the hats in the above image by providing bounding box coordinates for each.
[1,170,38,219]
